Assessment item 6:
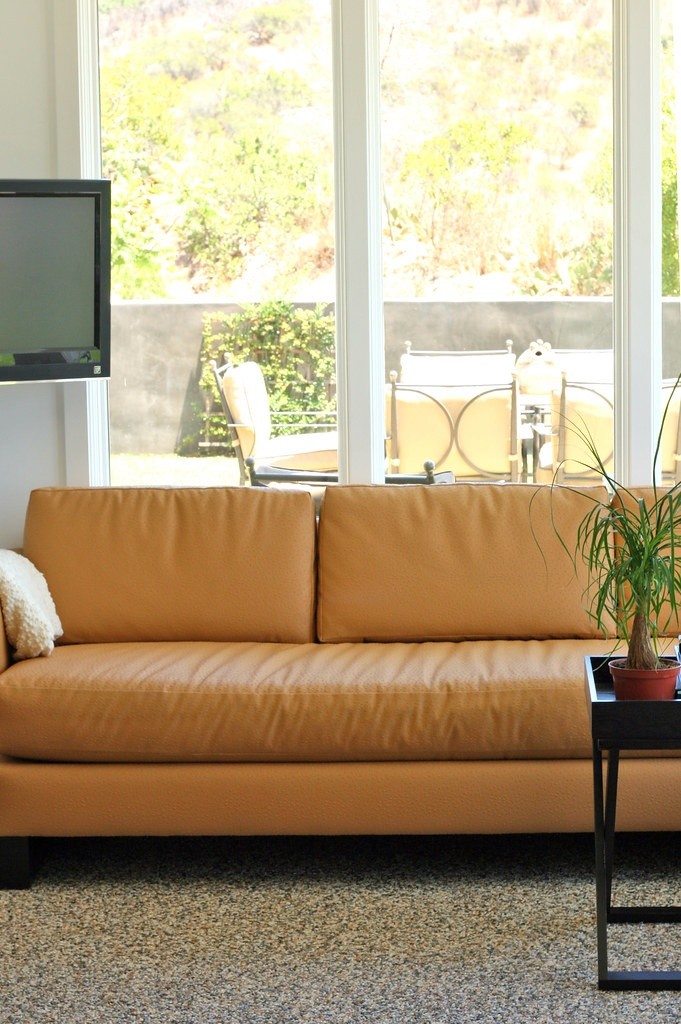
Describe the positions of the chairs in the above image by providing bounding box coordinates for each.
[518,347,615,382]
[399,339,517,384]
[529,373,681,485]
[384,368,527,484]
[211,352,338,487]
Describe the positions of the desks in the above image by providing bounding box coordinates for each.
[586,655,681,991]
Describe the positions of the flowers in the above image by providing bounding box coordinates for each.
[515,340,558,371]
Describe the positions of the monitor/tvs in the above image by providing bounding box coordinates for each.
[0,178,110,384]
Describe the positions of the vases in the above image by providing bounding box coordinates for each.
[517,369,556,395]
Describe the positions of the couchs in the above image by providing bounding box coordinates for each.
[0,483,681,835]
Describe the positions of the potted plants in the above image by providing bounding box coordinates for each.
[526,374,681,701]
